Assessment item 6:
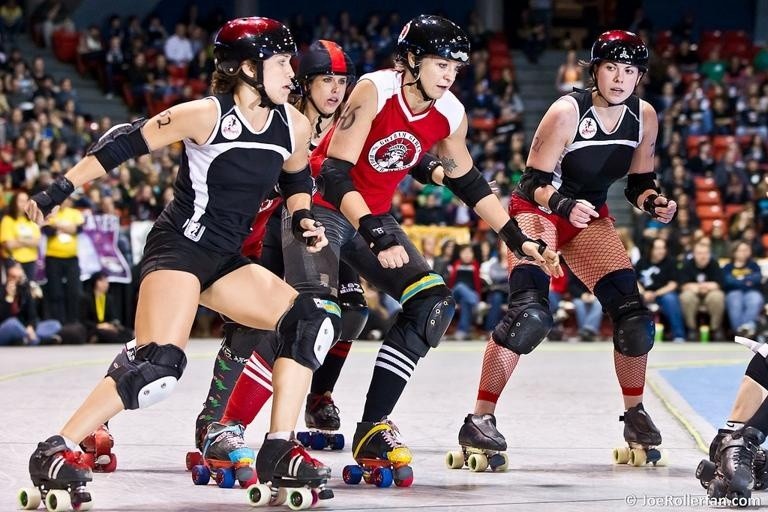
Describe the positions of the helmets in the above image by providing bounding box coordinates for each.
[590,29,648,72]
[293,39,356,79]
[397,13,471,66]
[213,15,297,60]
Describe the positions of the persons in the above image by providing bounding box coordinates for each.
[196,13,565,467]
[286,4,610,341]
[551,1,766,343]
[1,0,213,343]
[27,16,328,484]
[717,395,767,494]
[74,37,370,455]
[709,334,768,492]
[459,31,680,448]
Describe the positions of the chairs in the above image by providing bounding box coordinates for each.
[49,27,210,117]
[661,24,767,254]
[482,35,512,85]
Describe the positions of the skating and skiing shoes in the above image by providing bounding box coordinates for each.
[694,428,768,509]
[444,413,508,473]
[612,402,661,467]
[708,428,767,490]
[18,435,93,511]
[185,392,413,511]
[77,422,116,472]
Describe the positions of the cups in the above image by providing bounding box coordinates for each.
[650,323,664,341]
[699,325,711,343]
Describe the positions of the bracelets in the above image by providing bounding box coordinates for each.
[544,187,580,219]
